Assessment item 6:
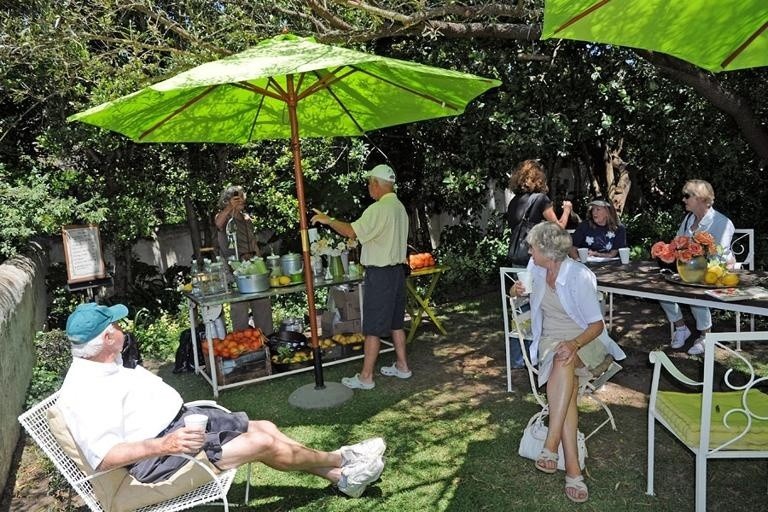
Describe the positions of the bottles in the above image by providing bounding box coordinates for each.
[190,256,231,297]
[227,256,266,275]
[348,261,357,278]
[280,250,304,276]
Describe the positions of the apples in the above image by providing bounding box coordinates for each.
[200,328,265,359]
[705,272,718,284]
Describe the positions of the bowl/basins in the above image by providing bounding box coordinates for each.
[233,271,270,294]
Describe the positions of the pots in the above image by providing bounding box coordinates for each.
[264,330,308,353]
[270,359,314,373]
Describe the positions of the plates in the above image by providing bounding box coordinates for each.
[270,282,305,289]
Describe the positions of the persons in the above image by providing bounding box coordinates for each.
[569,197,628,263]
[505,158,575,372]
[657,176,736,356]
[509,219,628,504]
[212,184,278,338]
[62,299,389,501]
[307,165,414,390]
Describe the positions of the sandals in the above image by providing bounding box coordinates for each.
[534,441,562,475]
[564,475,589,503]
[341,373,376,389]
[381,362,413,380]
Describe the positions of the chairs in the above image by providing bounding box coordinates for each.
[496,265,538,396]
[564,228,614,338]
[15,384,255,511]
[669,227,760,354]
[645,328,767,512]
[507,294,618,444]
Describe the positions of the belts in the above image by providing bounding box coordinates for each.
[155,400,187,441]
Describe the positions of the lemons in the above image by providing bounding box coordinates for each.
[279,276,290,284]
[721,274,739,286]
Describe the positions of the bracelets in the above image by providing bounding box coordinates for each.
[575,339,581,351]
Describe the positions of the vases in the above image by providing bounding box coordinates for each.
[675,252,708,283]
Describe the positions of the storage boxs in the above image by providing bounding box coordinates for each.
[318,281,361,343]
[503,328,537,370]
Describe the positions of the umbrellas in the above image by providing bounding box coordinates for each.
[538,0,768,78]
[61,23,505,387]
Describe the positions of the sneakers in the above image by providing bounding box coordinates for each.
[332,437,385,499]
[671,325,691,350]
[687,336,708,355]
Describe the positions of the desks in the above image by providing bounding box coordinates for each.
[399,263,453,344]
[180,263,396,401]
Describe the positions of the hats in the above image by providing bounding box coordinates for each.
[586,193,611,207]
[361,165,396,183]
[64,302,127,344]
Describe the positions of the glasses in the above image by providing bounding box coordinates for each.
[681,194,693,199]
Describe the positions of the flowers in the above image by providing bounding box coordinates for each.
[650,229,719,265]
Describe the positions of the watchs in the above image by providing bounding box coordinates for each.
[328,217,336,226]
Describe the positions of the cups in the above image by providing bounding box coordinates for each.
[617,247,629,265]
[183,414,207,435]
[205,317,227,341]
[577,248,588,263]
[266,255,280,271]
[515,272,533,294]
[289,274,303,282]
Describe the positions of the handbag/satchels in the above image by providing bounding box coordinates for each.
[173,324,206,373]
[508,192,541,266]
[516,403,588,473]
